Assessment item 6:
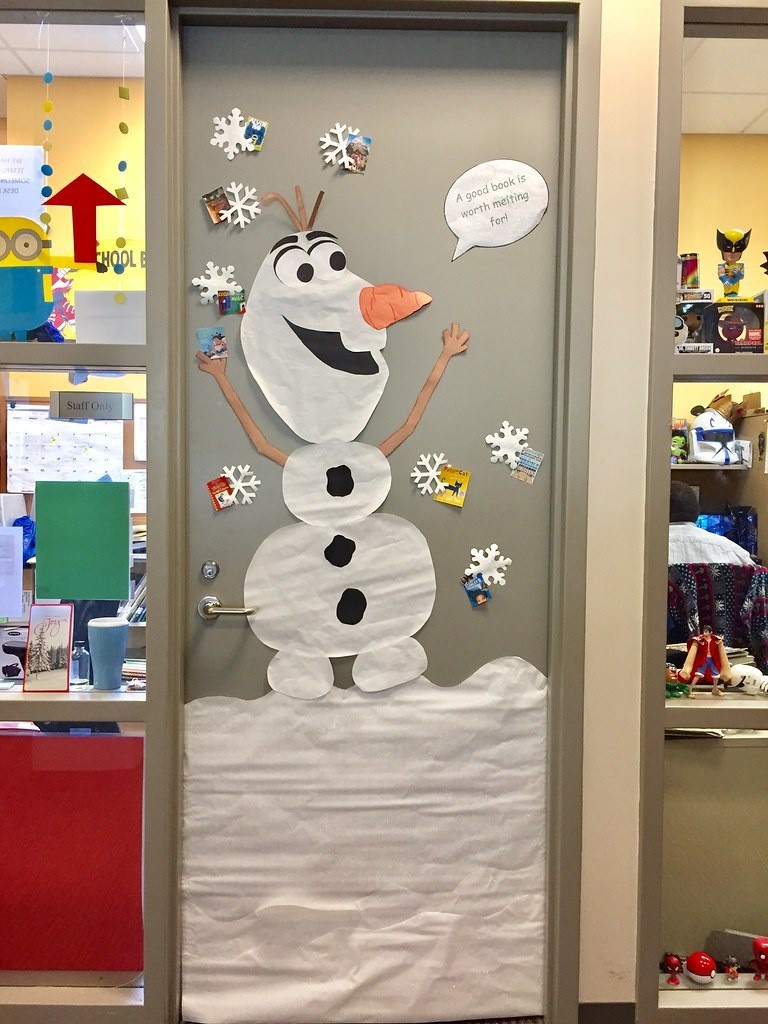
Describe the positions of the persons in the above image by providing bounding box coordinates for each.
[677,303,703,341]
[668,480,756,571]
[678,623,732,699]
[718,316,747,343]
[716,227,752,297]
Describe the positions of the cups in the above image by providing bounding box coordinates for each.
[88,617,129,689]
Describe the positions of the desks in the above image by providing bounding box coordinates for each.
[666,683,768,734]
[0,676,146,723]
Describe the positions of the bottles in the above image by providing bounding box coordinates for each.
[69,641,90,690]
